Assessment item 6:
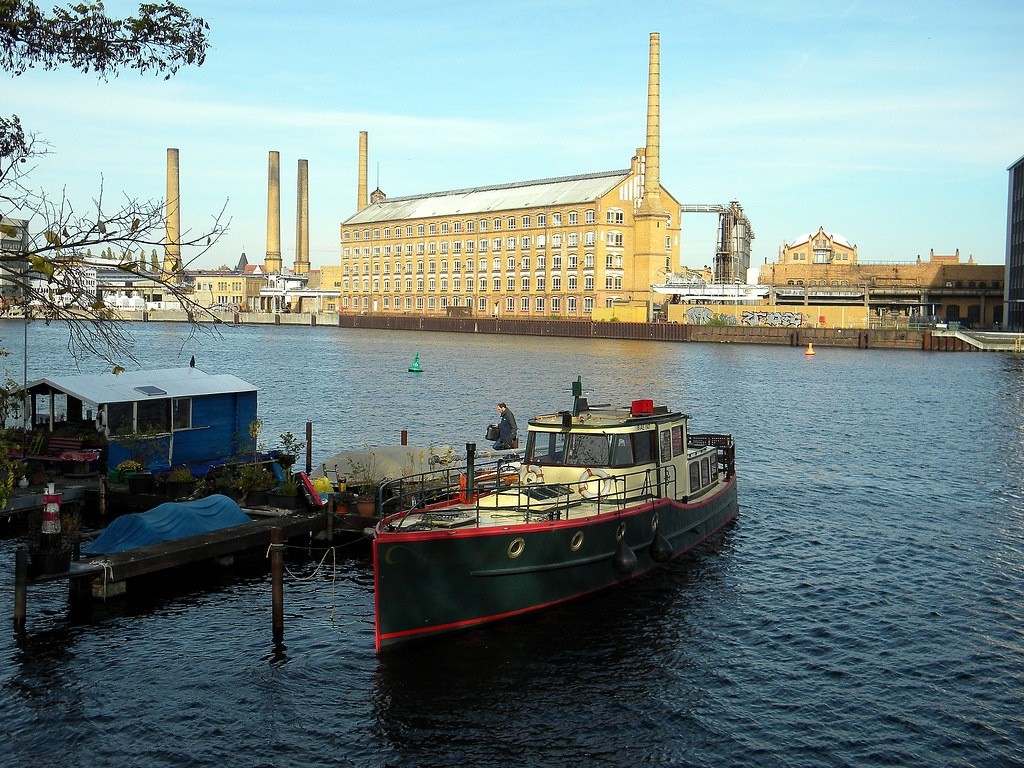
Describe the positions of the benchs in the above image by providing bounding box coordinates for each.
[26,437,81,475]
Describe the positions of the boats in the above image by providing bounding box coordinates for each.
[0,365,296,529]
[372,374,739,652]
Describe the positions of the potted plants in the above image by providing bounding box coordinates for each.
[334,494,348,514]
[166,464,197,498]
[203,476,243,503]
[241,463,274,506]
[16,462,29,488]
[273,468,297,510]
[357,472,375,517]
[342,443,375,493]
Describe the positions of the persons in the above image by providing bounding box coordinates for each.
[492,402,517,451]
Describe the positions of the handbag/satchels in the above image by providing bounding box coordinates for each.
[485,424,500,440]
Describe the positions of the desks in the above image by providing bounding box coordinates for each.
[59,448,102,475]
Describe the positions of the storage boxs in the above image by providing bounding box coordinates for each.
[632,400,653,415]
[653,406,668,415]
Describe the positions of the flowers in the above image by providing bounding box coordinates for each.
[115,460,140,469]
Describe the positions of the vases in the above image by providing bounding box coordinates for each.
[128,474,156,506]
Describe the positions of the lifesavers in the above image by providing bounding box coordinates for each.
[578,469,611,500]
[517,465,545,489]
